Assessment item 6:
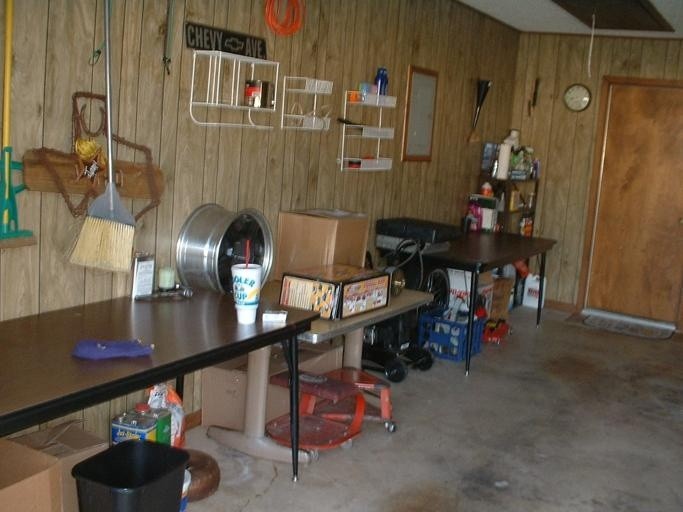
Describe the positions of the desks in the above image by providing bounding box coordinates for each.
[259,274,433,411]
[387,227,556,376]
[1,286,318,486]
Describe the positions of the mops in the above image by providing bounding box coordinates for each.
[0,0,38,248]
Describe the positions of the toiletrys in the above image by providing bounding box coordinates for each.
[376,66,388,95]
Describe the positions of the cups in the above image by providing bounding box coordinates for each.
[158,263,176,291]
[230,262,260,322]
[348,90,363,103]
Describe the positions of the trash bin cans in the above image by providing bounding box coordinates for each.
[72,439,190,512]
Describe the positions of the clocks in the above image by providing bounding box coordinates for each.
[562,84,590,112]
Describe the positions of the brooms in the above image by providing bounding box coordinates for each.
[69,0,136,275]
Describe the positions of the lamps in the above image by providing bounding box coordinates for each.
[468,78,492,144]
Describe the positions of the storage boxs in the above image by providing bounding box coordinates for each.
[490,273,513,320]
[19,423,108,511]
[279,265,391,320]
[274,206,367,284]
[199,341,344,434]
[0,436,62,512]
[446,268,491,318]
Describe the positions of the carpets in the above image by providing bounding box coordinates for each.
[563,310,675,340]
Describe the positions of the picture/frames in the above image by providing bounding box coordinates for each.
[400,63,438,163]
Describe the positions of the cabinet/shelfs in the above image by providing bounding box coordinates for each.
[482,171,540,309]
[280,73,334,132]
[338,88,397,172]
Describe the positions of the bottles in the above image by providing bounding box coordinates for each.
[521,189,534,217]
[374,68,388,107]
[357,83,378,105]
[480,180,491,195]
[442,291,485,326]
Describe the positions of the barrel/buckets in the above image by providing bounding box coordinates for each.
[110,400,171,449]
[521,272,547,308]
